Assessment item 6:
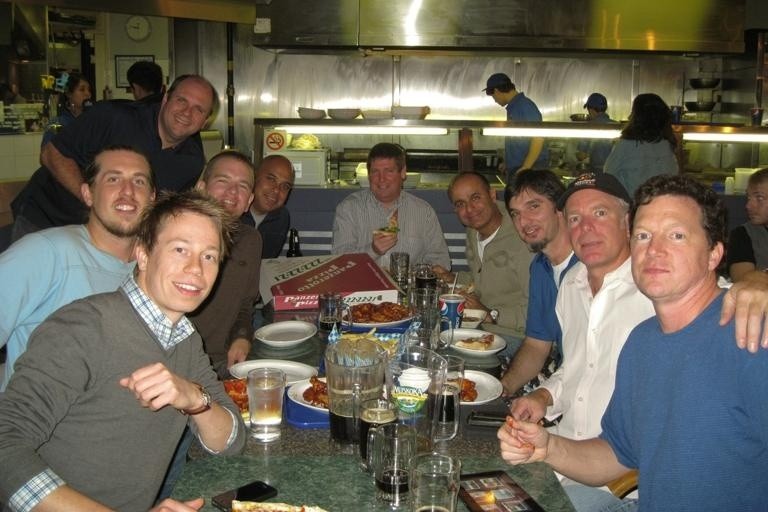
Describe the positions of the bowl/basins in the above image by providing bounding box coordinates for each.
[689,78,720,88]
[570,114,590,121]
[685,101,718,112]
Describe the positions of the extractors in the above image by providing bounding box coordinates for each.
[251,1,763,58]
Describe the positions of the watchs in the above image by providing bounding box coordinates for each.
[179,382,212,415]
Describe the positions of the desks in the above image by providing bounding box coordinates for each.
[156,290,577,511]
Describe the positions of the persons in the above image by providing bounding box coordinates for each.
[11,74,215,245]
[0,143,157,393]
[127,61,163,100]
[42,74,92,147]
[499,169,581,405]
[0,189,247,512]
[575,93,618,169]
[604,94,679,198]
[430,171,539,357]
[331,143,453,272]
[183,152,264,380]
[482,73,546,183]
[239,155,294,258]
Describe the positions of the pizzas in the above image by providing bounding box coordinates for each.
[373,208,398,236]
[456,335,494,351]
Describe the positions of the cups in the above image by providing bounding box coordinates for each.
[724,177,735,194]
[390,252,408,281]
[671,105,683,123]
[246,366,286,442]
[750,108,765,127]
[318,263,463,512]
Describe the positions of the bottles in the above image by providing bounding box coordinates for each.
[287,228,303,257]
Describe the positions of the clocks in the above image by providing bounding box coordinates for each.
[124,16,151,43]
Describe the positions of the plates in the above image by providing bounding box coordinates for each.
[254,343,320,359]
[327,109,360,119]
[448,282,470,295]
[296,107,327,119]
[254,319,319,348]
[288,377,328,413]
[438,327,508,355]
[362,110,394,119]
[461,307,487,328]
[389,104,430,121]
[355,174,371,188]
[457,368,504,407]
[402,173,421,188]
[229,359,318,385]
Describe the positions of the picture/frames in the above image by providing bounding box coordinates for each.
[114,54,156,90]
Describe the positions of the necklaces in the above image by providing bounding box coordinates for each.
[727,167,768,282]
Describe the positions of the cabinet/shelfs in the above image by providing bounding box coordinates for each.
[250,116,768,272]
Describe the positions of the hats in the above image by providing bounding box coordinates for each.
[482,74,510,91]
[556,172,631,211]
[584,93,606,108]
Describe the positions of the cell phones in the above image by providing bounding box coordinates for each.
[212,480,277,512]
[468,411,506,428]
